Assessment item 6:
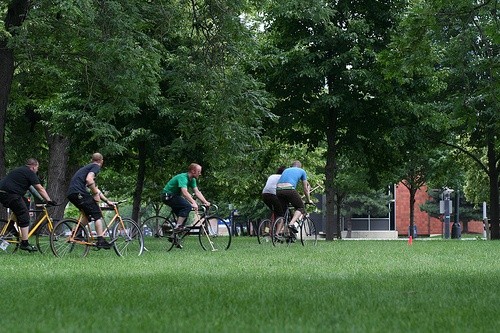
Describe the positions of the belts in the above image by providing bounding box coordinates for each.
[276,187,295,190]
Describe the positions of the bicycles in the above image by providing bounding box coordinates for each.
[138,203,232,252]
[257,201,317,249]
[0,202,76,258]
[49,200,144,260]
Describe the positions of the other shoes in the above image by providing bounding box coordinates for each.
[275,234,281,240]
[168,236,183,249]
[97,238,113,247]
[20,245,38,251]
[76,232,84,239]
[288,224,298,233]
[174,225,186,233]
[7,225,18,237]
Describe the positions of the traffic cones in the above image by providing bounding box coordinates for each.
[407,235,413,246]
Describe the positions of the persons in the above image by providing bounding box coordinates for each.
[162,163,210,248]
[67,153,119,249]
[274,160,313,241]
[263,165,286,235]
[0,158,57,251]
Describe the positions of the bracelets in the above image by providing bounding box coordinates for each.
[94,192,98,194]
